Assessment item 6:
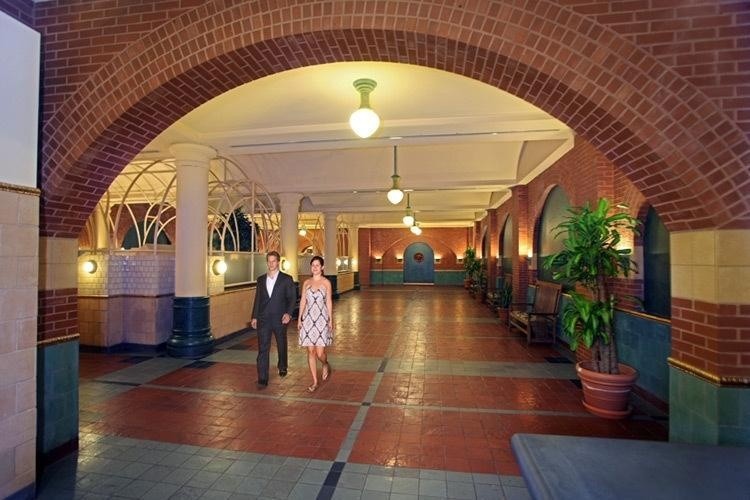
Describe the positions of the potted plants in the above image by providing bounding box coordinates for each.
[496,280,512,322]
[538,194,642,418]
[476,261,486,304]
[463,248,479,289]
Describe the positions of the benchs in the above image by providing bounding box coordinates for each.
[506,279,565,347]
[487,272,504,312]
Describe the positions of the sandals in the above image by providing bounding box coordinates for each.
[321,363,331,382]
[305,383,319,393]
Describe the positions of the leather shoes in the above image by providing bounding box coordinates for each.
[279,369,287,376]
[256,379,268,388]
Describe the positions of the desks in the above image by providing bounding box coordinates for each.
[510,431,750,500]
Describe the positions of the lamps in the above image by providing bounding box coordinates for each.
[403,192,415,228]
[417,223,421,237]
[346,78,380,138]
[281,260,291,270]
[213,259,227,275]
[386,145,404,205]
[84,261,97,276]
[411,215,418,233]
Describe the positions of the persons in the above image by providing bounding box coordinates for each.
[297,256,333,391]
[251,251,297,387]
